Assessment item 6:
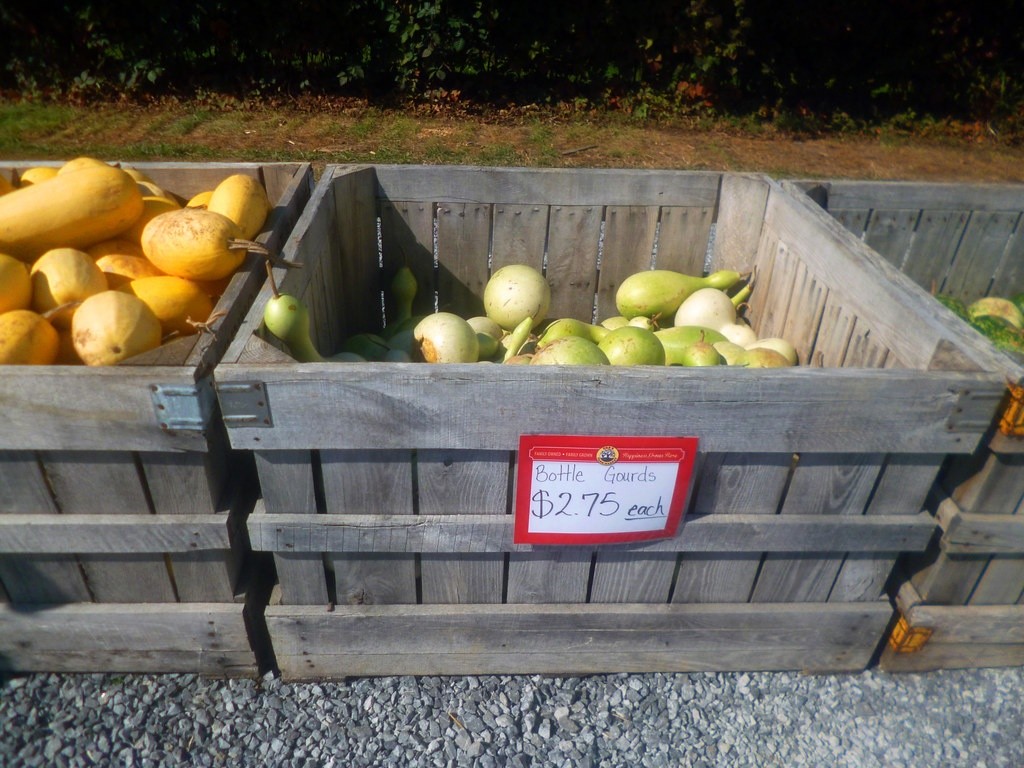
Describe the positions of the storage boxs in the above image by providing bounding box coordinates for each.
[0,160,1024,681]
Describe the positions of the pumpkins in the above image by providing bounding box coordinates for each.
[0,157,272,367]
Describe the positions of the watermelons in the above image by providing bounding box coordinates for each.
[932,294,1024,355]
